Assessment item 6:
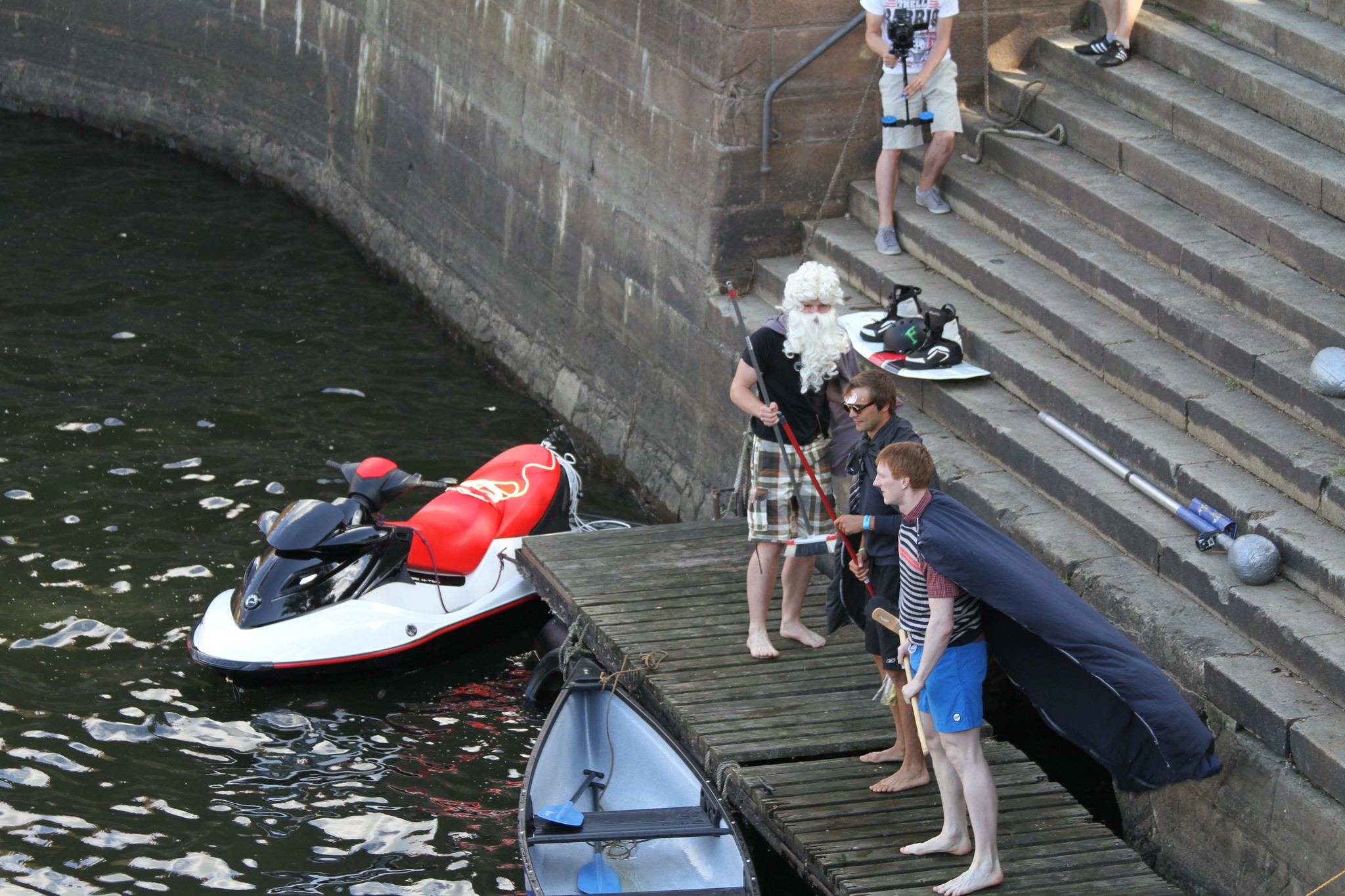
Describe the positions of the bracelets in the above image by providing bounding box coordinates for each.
[863,515,871,530]
[858,548,867,552]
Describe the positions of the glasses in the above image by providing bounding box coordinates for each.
[842,400,876,415]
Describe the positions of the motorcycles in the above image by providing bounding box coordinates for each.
[187,423,585,679]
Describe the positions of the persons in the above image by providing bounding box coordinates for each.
[1073,0,1146,66]
[858,0,963,256]
[831,370,941,794]
[730,258,859,659]
[871,441,1004,895]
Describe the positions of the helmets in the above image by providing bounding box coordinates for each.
[883,317,929,354]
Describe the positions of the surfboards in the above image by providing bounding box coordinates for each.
[837,311,990,381]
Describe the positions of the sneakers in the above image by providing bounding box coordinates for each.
[1095,40,1131,67]
[874,224,902,255]
[915,186,951,214]
[904,303,964,370]
[1074,34,1110,55]
[860,284,922,343]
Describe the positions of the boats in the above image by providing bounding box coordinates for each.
[515,657,760,896]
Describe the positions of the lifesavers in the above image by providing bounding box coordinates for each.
[521,645,595,703]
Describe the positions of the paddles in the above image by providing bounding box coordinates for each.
[577,782,623,895]
[537,769,604,826]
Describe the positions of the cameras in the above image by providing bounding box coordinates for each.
[886,19,929,45]
